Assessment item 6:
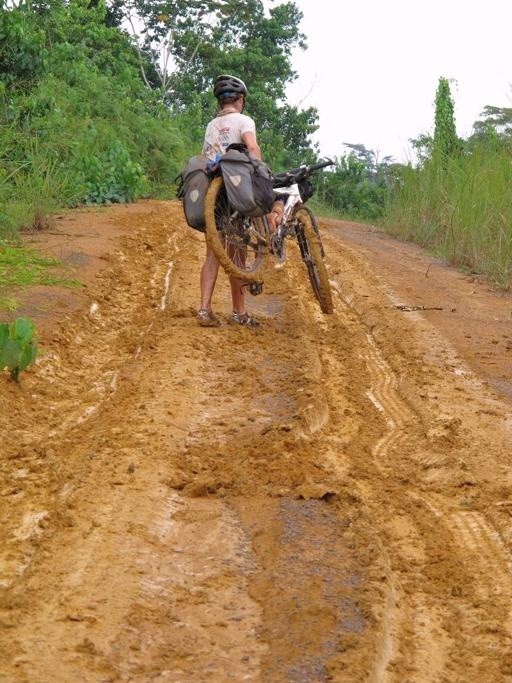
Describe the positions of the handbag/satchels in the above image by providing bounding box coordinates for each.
[217,149,277,220]
[282,177,317,207]
[176,154,227,233]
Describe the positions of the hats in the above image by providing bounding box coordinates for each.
[213,73,249,99]
[197,309,222,327]
[230,310,262,329]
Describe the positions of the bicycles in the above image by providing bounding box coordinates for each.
[202,157,340,316]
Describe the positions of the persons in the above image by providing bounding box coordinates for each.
[196,73,261,328]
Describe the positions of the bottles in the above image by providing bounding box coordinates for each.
[270,198,284,223]
